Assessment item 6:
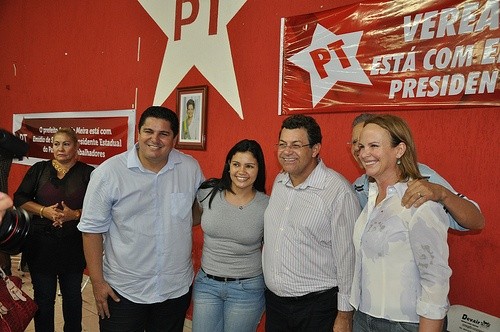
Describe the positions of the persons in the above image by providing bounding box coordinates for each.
[348,114,451,332]
[182,99,198,140]
[77,106,206,332]
[192,139,271,332]
[262,113,362,332]
[351,114,485,231]
[0,125,96,332]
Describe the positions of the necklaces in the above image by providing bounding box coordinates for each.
[53,159,76,173]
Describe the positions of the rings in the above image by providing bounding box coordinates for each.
[417,192,423,198]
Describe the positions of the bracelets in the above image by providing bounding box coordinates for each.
[40,206,45,219]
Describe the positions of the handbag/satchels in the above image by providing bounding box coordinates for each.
[0,267,39,332]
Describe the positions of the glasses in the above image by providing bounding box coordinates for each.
[347,138,359,148]
[275,143,310,153]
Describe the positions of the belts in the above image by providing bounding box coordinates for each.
[264,285,339,303]
[201,267,255,282]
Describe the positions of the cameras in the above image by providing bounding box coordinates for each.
[0,207,34,255]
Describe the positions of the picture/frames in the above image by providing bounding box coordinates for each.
[176,84,209,151]
